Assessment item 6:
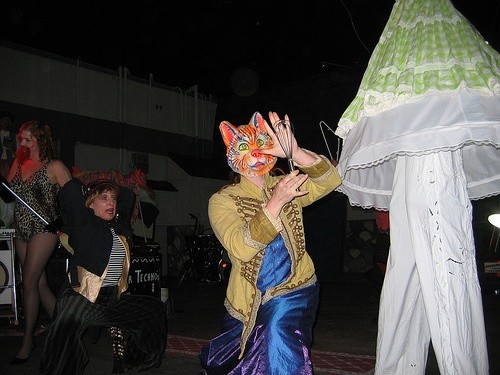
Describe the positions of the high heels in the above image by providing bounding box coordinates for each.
[11,341,36,365]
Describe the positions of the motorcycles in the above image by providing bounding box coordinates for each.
[174,212,232,289]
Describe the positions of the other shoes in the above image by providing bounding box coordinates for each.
[86,326,101,343]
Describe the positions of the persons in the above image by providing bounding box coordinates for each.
[40,170,169,375]
[0,120,72,365]
[199,111,342,375]
[333,0,500,375]
[363,210,393,325]
